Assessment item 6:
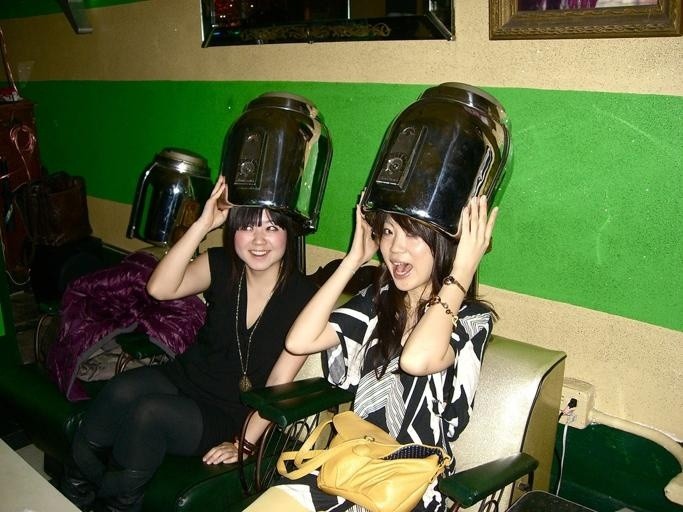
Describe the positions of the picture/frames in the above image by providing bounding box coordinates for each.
[488,0,682,38]
[198,0,455,48]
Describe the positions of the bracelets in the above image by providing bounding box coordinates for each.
[430,295,458,327]
[442,276,466,296]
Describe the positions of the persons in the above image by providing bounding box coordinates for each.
[241,185,499,511]
[60,173,324,508]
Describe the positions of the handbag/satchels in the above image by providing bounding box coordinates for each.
[277,411,449,512]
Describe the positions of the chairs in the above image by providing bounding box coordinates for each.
[118,283,326,510]
[263,332,565,510]
[2,236,131,474]
